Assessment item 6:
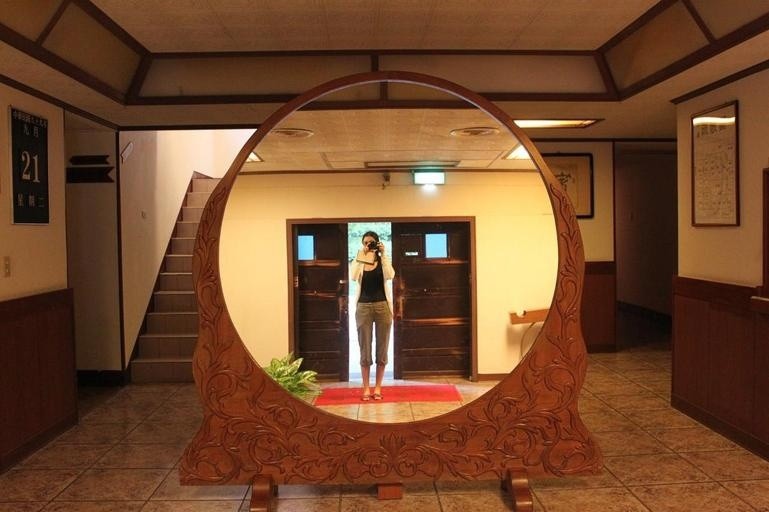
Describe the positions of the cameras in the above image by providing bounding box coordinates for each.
[367,241,377,250]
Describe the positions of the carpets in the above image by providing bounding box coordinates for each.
[310,383,463,407]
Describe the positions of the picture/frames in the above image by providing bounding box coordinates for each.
[690,99,741,228]
[538,152,594,219]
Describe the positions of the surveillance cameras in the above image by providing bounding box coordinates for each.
[382,171,391,182]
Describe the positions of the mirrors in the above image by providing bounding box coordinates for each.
[168,68,607,488]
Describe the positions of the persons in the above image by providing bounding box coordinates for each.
[350,231,396,401]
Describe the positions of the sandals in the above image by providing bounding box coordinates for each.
[362,392,382,401]
